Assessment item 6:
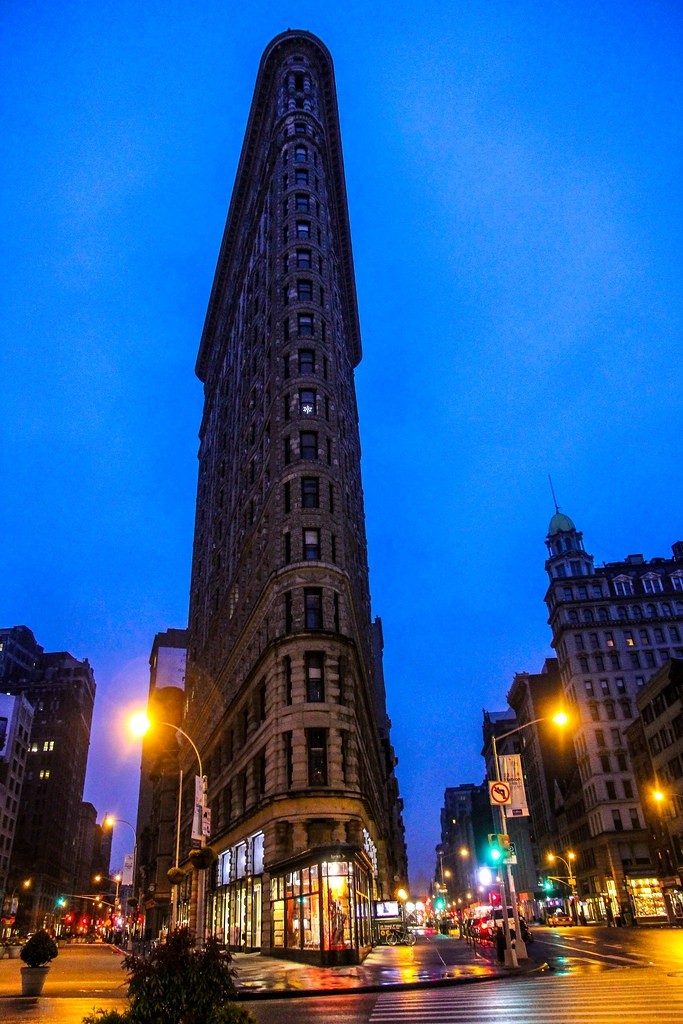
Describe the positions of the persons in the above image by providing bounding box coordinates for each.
[519,915,531,944]
[494,927,516,966]
[675,903,683,928]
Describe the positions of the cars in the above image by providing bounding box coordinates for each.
[547,908,574,927]
[469,918,498,941]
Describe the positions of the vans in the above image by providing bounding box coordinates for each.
[474,905,526,944]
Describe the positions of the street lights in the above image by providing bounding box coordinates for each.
[438,848,468,934]
[491,711,568,958]
[124,708,205,954]
[94,875,118,913]
[647,788,683,804]
[396,887,407,922]
[11,881,30,899]
[546,851,581,927]
[105,817,136,950]
[477,865,521,970]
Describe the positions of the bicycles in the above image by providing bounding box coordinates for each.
[385,925,417,946]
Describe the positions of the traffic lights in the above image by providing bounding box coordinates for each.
[55,896,63,908]
[488,892,499,905]
[498,834,511,860]
[488,834,502,867]
